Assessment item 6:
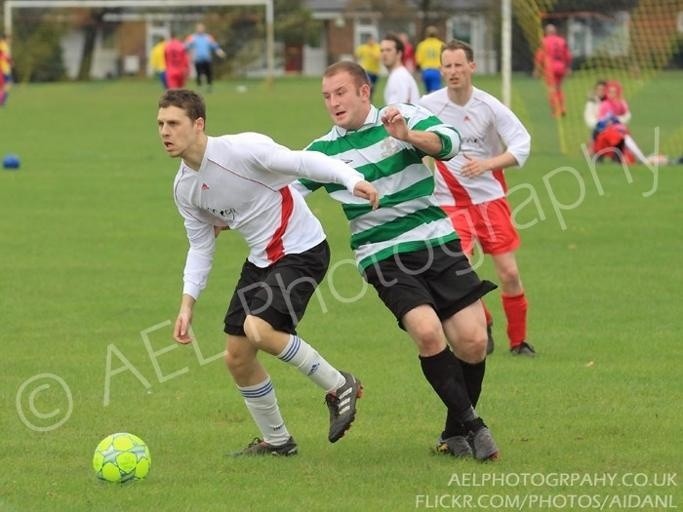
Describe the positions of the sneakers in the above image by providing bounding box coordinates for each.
[325,370,363,443]
[487,322,494,354]
[230,436,298,457]
[510,341,536,355]
[436,426,498,462]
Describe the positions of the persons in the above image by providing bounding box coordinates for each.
[419,40,533,358]
[1,36,13,107]
[533,25,636,165]
[215,62,499,464]
[184,22,227,90]
[157,89,379,459]
[357,26,444,102]
[150,36,167,87]
[164,30,190,88]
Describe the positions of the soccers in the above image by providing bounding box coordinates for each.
[93,433,151,484]
